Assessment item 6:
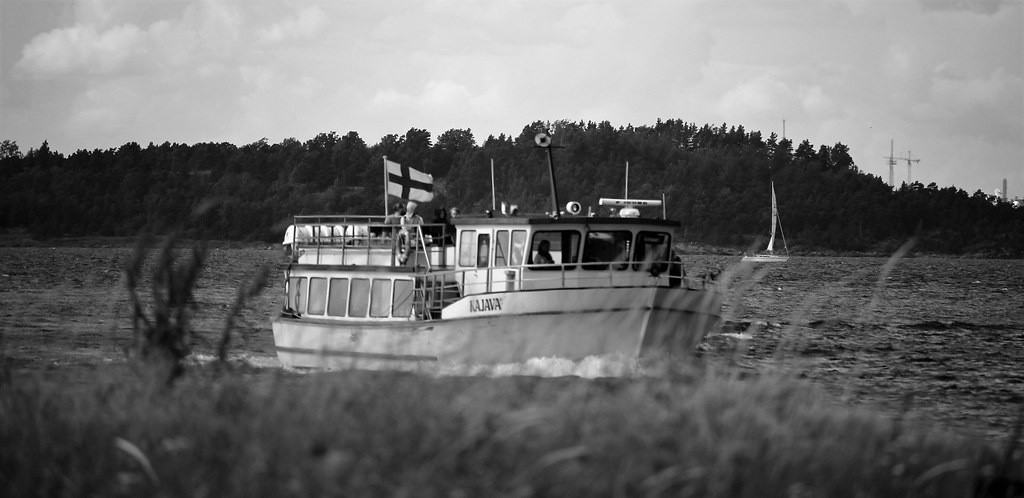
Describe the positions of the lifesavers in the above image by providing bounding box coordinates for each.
[395,228,411,264]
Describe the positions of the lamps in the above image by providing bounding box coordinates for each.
[566,201,581,216]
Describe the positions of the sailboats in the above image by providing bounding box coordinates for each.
[740,181,791,263]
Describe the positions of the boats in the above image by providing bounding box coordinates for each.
[273,130,724,377]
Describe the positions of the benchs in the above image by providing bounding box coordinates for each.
[296,244,455,267]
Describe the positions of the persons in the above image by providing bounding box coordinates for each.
[382,203,406,243]
[428,206,461,244]
[395,201,425,264]
[533,239,555,271]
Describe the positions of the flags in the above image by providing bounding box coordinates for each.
[386,159,434,203]
